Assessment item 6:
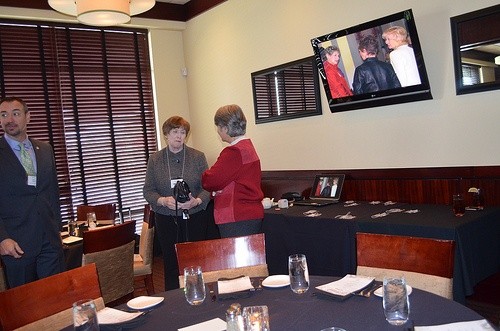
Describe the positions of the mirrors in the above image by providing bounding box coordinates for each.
[251,55,323,124]
[450,4,500,96]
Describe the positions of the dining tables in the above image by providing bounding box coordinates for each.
[61,220,111,265]
[60,276,500,331]
[261,198,500,303]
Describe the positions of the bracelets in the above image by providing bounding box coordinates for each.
[162,200,165,207]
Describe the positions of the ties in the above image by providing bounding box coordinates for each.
[19,143,36,177]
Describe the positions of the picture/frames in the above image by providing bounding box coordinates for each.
[310,174,346,199]
[310,9,433,113]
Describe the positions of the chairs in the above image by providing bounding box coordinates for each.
[0,201,457,331]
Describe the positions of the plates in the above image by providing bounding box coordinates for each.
[375,284,414,296]
[128,295,165,312]
[62,236,83,244]
[263,274,291,289]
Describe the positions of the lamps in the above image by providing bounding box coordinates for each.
[47,0,155,26]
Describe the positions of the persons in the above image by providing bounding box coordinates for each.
[331,179,338,197]
[321,180,331,196]
[144,116,210,293]
[382,27,421,87]
[323,46,352,99]
[202,104,264,238]
[351,35,401,96]
[0,98,64,290]
[315,179,323,196]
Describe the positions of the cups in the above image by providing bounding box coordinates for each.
[86,212,96,230]
[382,274,410,325]
[73,299,100,331]
[453,192,465,216]
[183,266,207,304]
[468,191,481,209]
[320,327,347,331]
[68,219,79,237]
[288,254,309,294]
[243,305,270,331]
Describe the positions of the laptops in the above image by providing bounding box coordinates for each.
[293,174,346,206]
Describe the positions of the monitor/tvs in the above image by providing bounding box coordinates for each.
[311,8,433,113]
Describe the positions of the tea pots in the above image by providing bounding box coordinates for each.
[261,196,274,209]
[278,198,289,209]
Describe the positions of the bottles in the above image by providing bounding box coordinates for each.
[226,302,245,331]
